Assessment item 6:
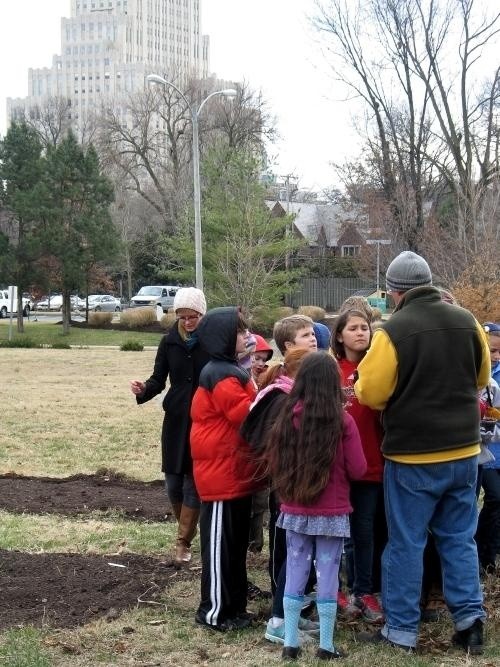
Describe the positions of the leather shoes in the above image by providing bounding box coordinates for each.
[353,627,415,655]
[281,643,305,661]
[451,623,486,654]
[316,646,347,663]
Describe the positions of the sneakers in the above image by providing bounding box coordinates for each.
[350,591,386,626]
[247,580,271,602]
[264,615,317,649]
[299,594,318,618]
[193,602,258,633]
[334,591,365,624]
[298,616,322,635]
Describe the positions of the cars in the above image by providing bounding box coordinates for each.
[37,294,121,312]
[0,289,34,317]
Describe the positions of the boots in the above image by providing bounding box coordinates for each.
[170,500,201,565]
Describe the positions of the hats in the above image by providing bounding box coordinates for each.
[173,287,208,318]
[384,249,434,292]
[482,321,500,332]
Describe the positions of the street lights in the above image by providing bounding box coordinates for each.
[147,74,237,289]
[366,239,391,307]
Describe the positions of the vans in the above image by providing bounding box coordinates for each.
[131,285,180,309]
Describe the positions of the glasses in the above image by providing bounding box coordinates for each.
[175,314,201,323]
[386,288,407,295]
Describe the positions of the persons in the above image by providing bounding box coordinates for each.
[131,250,500,663]
